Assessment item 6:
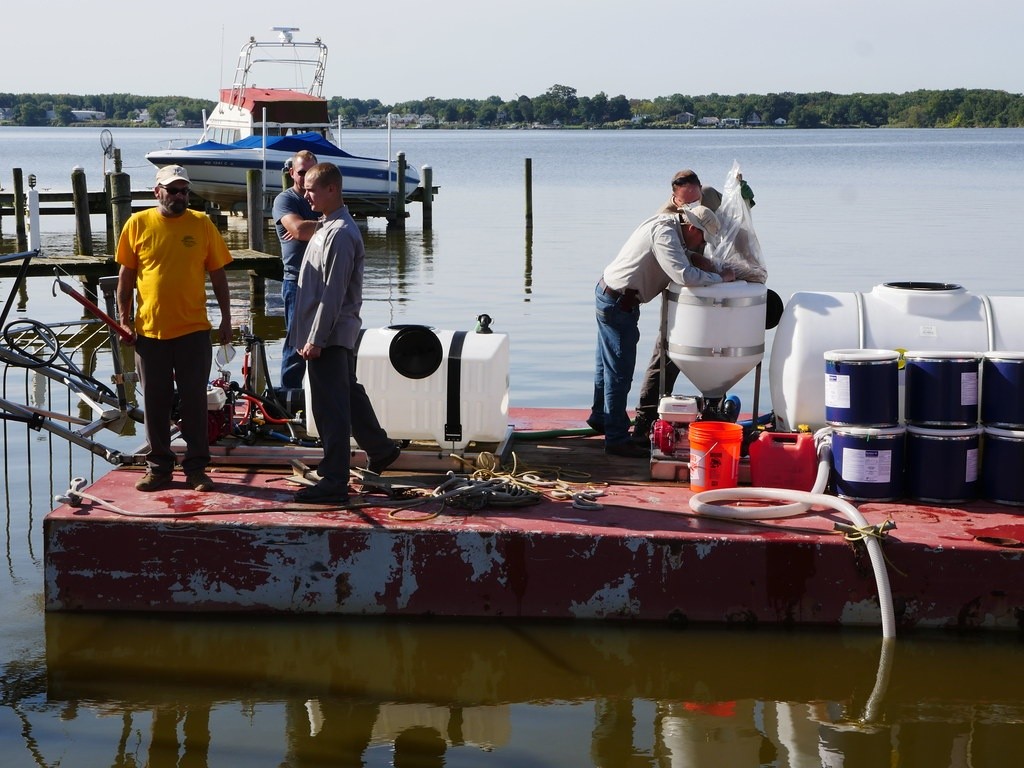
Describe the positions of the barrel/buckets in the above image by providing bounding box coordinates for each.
[687,421,744,494]
[903,350,984,426]
[903,424,982,504]
[981,426,1024,507]
[981,351,1024,428]
[823,348,901,427]
[824,420,904,503]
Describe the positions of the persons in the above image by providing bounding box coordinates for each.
[287,161,400,501]
[273,151,348,441]
[632,169,755,442]
[115,167,236,491]
[587,204,716,455]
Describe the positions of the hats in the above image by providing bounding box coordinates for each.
[155,165,192,186]
[677,205,720,249]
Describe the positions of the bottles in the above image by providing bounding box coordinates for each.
[747,430,817,493]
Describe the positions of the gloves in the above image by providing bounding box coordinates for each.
[740,180,754,200]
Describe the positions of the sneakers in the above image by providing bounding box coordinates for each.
[134,472,173,490]
[367,444,400,475]
[186,474,215,492]
[293,478,349,502]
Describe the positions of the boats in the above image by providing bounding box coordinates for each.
[144,27,422,235]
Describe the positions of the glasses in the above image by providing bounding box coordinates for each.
[292,167,306,176]
[159,187,191,196]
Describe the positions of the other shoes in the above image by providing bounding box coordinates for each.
[586,417,606,434]
[604,441,647,458]
[632,431,651,448]
